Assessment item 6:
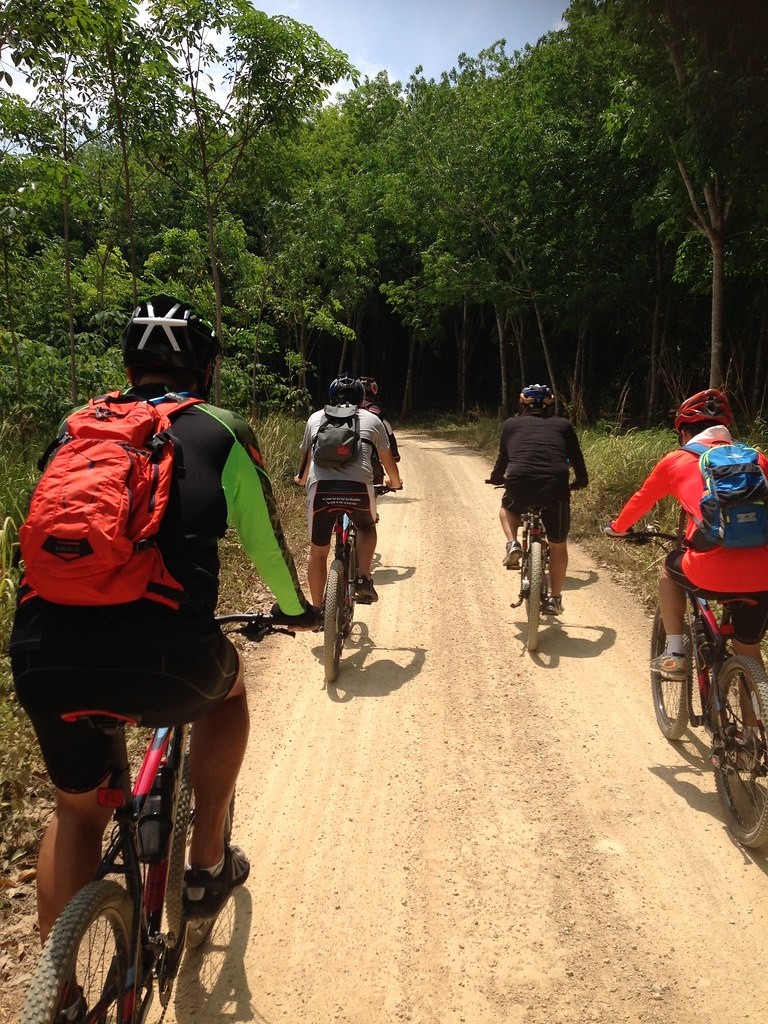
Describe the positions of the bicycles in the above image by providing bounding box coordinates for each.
[484,478,586,651]
[18,612,324,1024]
[604,524,768,848]
[292,481,403,683]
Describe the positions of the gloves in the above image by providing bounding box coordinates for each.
[603,525,633,542]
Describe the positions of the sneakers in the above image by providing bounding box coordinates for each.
[501,540,523,567]
[547,595,565,614]
[351,576,379,603]
[183,846,249,920]
[651,652,689,681]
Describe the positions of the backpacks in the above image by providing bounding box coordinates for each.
[678,442,768,547]
[18,390,204,616]
[311,401,374,468]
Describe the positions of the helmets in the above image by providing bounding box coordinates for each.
[358,377,378,397]
[674,389,732,433]
[123,292,221,364]
[328,372,365,399]
[519,384,555,405]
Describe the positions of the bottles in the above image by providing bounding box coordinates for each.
[137,755,167,862]
[694,614,715,670]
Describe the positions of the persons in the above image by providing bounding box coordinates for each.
[8,295,323,1024]
[490,383,589,616]
[294,372,403,631]
[357,377,400,499]
[604,389,768,771]
